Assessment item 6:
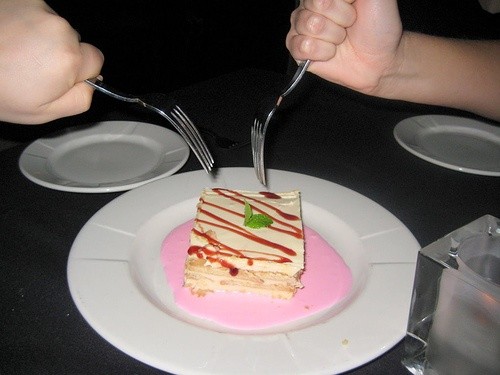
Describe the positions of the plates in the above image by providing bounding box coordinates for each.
[393,114,500,177]
[67,168,422,375]
[18,120,190,193]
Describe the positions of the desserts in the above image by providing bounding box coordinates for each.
[184,188,305,299]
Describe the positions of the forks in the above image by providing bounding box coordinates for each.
[84,78,215,175]
[250,60,311,186]
[197,127,250,149]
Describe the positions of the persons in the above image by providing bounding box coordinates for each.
[0,0,500,125]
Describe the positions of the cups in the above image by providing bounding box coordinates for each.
[399,212,500,375]
